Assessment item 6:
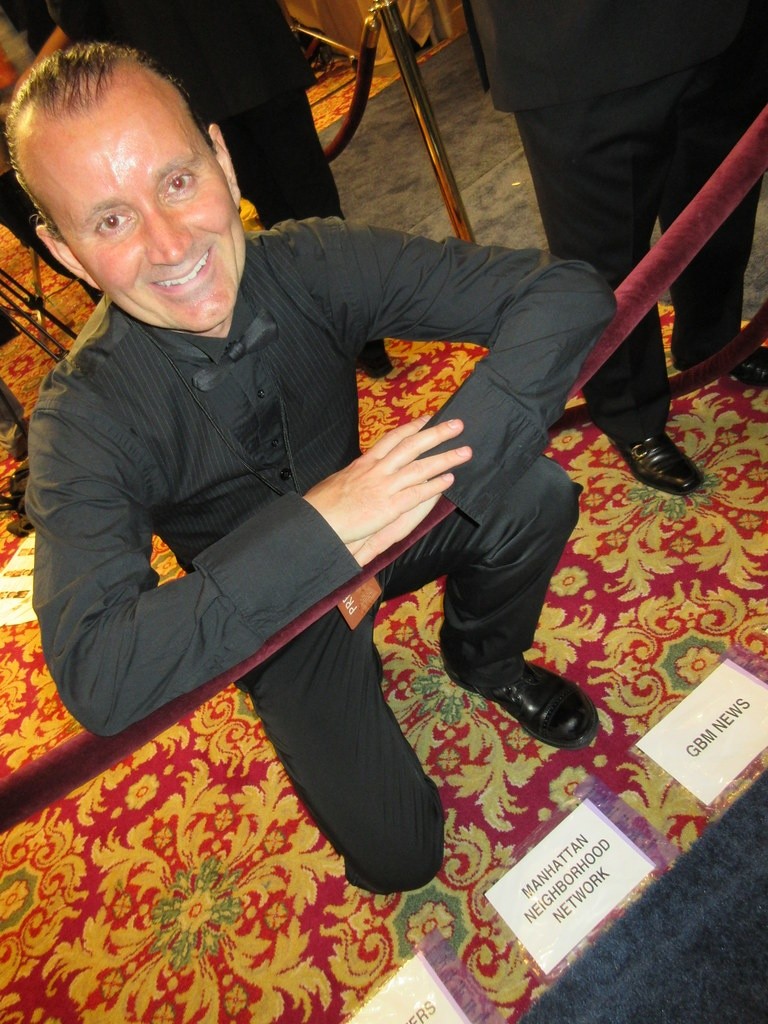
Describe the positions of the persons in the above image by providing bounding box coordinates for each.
[15,0,398,379]
[5,42,620,893]
[464,0,768,495]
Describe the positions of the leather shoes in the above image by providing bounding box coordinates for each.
[674,340,768,390]
[606,432,702,495]
[444,659,599,748]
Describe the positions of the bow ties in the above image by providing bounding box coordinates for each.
[192,308,277,392]
